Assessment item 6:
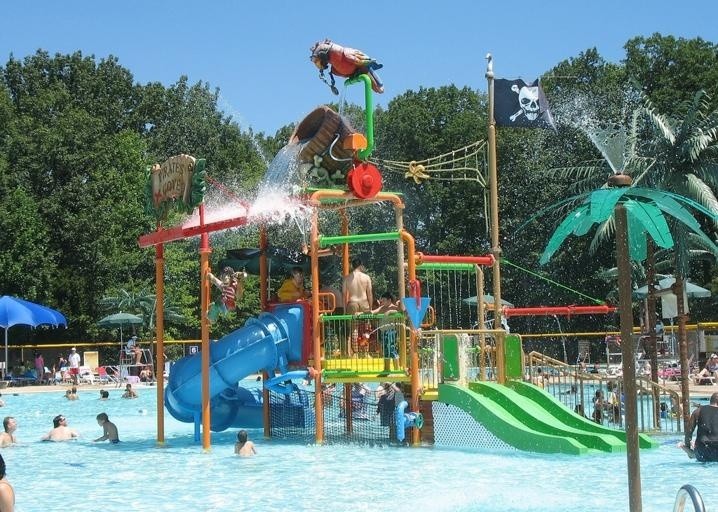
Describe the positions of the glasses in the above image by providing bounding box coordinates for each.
[61,417,65,422]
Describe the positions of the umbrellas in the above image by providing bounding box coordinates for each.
[0,295,67,374]
[98,310,141,350]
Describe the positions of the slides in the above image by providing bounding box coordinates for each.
[165,304,304,431]
[439,380,659,455]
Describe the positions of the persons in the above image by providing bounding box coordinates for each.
[40,415,78,442]
[0,456,17,512]
[5,335,168,401]
[92,413,120,444]
[234,430,257,456]
[0,393,6,407]
[0,417,18,450]
[203,266,247,323]
[254,258,718,464]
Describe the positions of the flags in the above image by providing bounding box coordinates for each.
[491,77,558,134]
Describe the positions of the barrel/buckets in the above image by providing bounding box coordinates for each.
[289,106,361,183]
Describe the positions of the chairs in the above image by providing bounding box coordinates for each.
[123,346,137,364]
[658,352,718,386]
[0,364,141,387]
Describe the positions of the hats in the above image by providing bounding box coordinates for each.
[70,348,76,351]
[131,335,139,339]
